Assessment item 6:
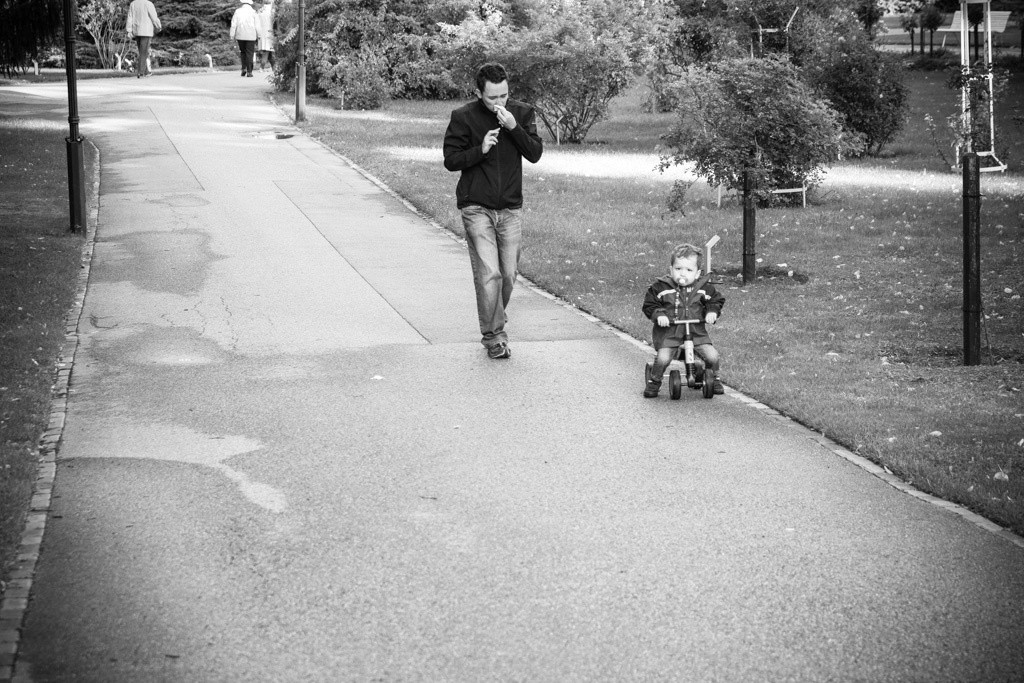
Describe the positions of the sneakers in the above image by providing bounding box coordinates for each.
[489,344,511,358]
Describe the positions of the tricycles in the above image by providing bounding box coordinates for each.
[644,318,717,401]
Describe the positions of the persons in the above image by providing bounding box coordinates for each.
[126,0,161,78]
[230,0,276,77]
[443,62,542,358]
[642,243,725,398]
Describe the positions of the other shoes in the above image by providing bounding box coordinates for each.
[712,377,724,394]
[644,380,660,397]
[137,72,151,78]
[242,73,252,78]
